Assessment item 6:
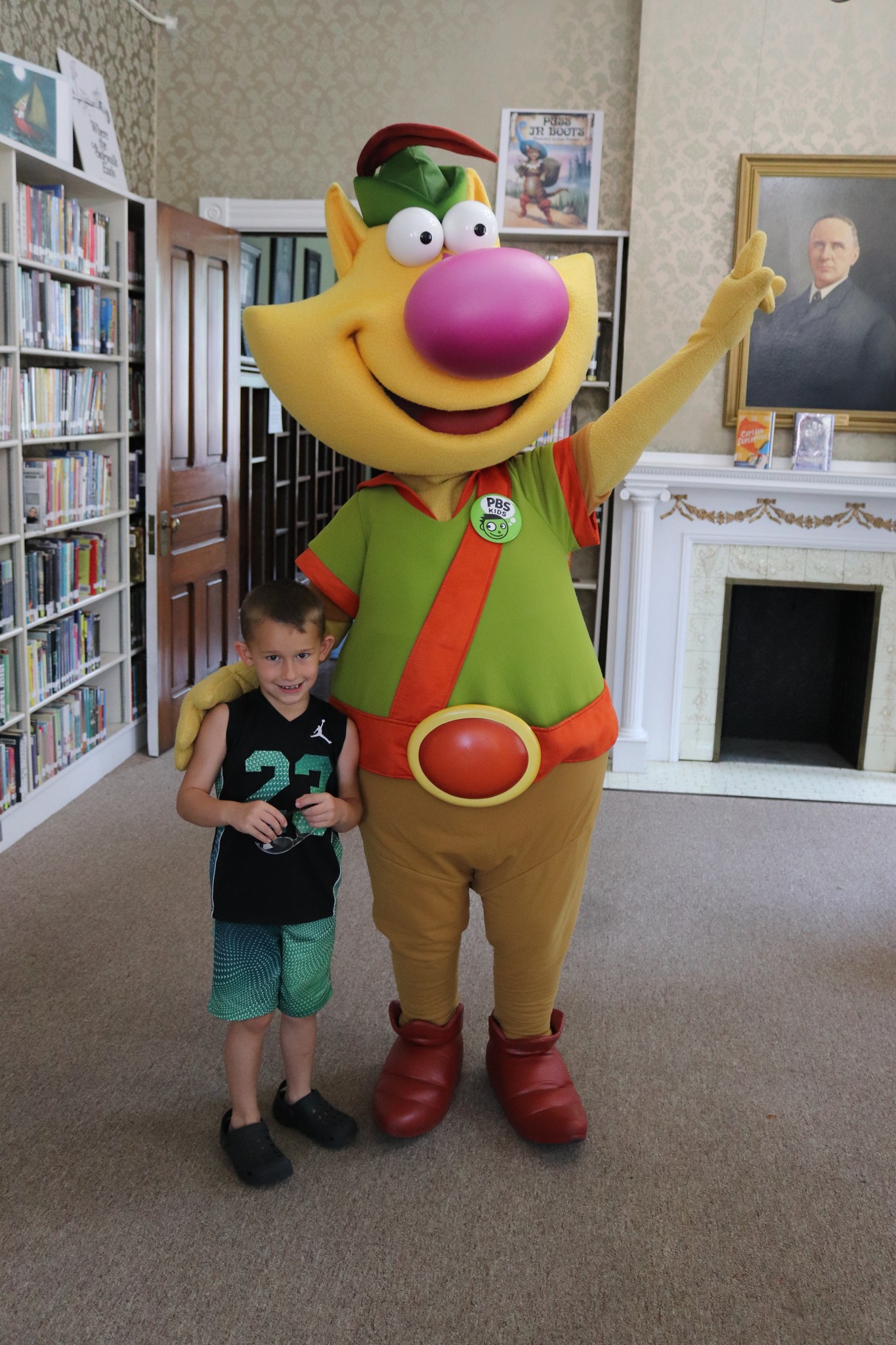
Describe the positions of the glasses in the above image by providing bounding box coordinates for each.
[255,817,316,855]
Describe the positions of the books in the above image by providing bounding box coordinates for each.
[0,177,146,811]
[586,320,605,383]
[525,400,572,453]
[791,411,835,470]
[735,407,777,469]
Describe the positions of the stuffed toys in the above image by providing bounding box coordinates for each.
[171,118,782,1150]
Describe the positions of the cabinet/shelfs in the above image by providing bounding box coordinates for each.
[241,367,367,613]
[496,228,631,656]
[0,135,147,854]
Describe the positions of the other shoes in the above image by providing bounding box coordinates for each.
[272,1079,358,1148]
[220,1108,293,1187]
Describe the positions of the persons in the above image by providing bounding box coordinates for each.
[173,586,363,1196]
[750,214,895,411]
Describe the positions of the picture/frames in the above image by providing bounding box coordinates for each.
[269,236,297,304]
[241,240,262,367]
[722,153,896,432]
[496,108,604,230]
[303,246,322,299]
[0,49,74,165]
